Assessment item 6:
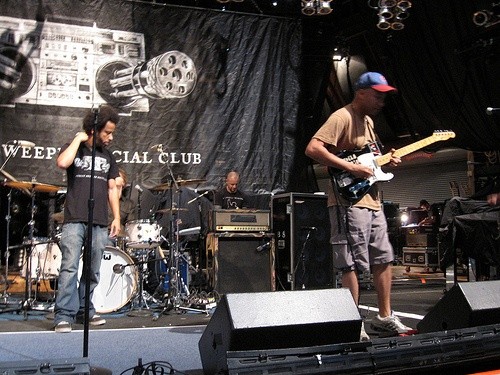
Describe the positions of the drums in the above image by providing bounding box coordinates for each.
[123,218,162,249]
[77,245,140,313]
[19,235,63,279]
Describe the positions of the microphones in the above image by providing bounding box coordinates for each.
[74,131,93,152]
[256,242,270,253]
[113,263,124,274]
[299,225,317,230]
[134,184,144,193]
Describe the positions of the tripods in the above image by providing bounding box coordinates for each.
[0,144,213,315]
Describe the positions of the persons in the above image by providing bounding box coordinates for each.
[54,105,121,333]
[305,73,417,341]
[214,172,249,209]
[417,200,440,226]
[52,168,135,254]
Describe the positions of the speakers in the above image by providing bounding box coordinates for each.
[205,234,275,297]
[197,286,363,375]
[270,193,340,290]
[416,279,500,334]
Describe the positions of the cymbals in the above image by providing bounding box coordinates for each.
[154,207,191,214]
[153,178,208,190]
[4,180,63,193]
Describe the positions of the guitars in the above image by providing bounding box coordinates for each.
[330,129,456,201]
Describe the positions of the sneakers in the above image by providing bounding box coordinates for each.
[76,313,105,326]
[54,320,72,332]
[372,311,413,335]
[360,322,369,342]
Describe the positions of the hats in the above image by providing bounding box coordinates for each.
[354,72,397,92]
